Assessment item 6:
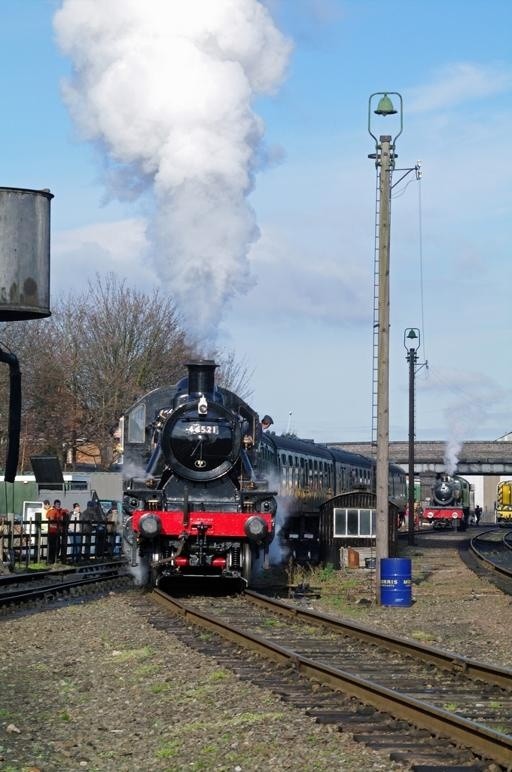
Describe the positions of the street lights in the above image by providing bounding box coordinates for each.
[368,89,429,603]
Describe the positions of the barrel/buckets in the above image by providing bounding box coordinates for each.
[380,557,411,606]
[367,559,376,569]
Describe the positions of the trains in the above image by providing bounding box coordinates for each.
[112,357,408,580]
[496,480,512,528]
[407,468,473,530]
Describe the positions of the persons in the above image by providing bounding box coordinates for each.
[41,499,52,558]
[81,501,98,561]
[417,503,424,526]
[105,506,123,560]
[46,500,68,565]
[475,504,483,525]
[68,503,82,563]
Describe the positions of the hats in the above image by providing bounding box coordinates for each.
[264,415,273,424]
